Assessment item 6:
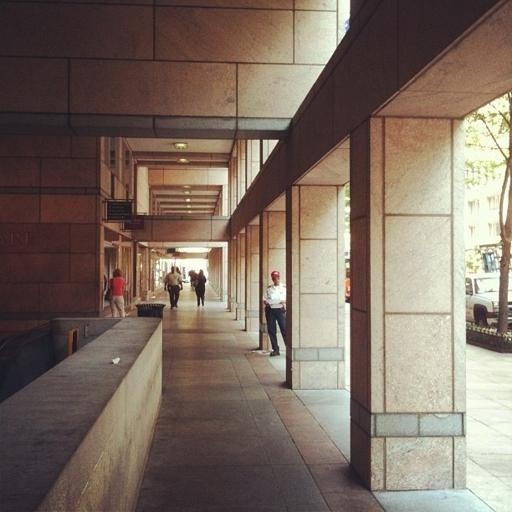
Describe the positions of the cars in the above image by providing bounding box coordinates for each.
[465,272,512,329]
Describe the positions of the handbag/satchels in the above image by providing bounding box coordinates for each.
[105,279,113,300]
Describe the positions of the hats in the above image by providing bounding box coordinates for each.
[272,271,279,278]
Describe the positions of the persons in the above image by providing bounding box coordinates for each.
[163,266,183,310]
[109,268,126,317]
[195,269,207,306]
[263,271,287,357]
[176,267,181,274]
[190,270,196,291]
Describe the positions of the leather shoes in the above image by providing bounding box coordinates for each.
[271,351,279,356]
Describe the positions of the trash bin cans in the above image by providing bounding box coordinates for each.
[135,304,165,317]
